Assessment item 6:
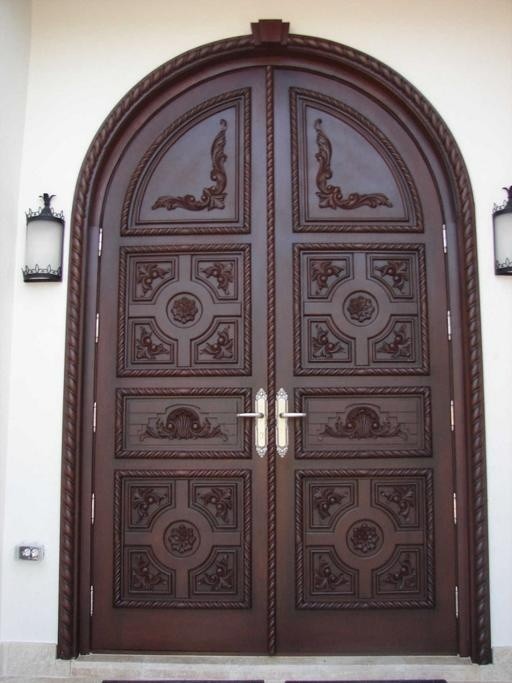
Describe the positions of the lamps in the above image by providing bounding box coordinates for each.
[491,185,512,276]
[20,192,65,284]
